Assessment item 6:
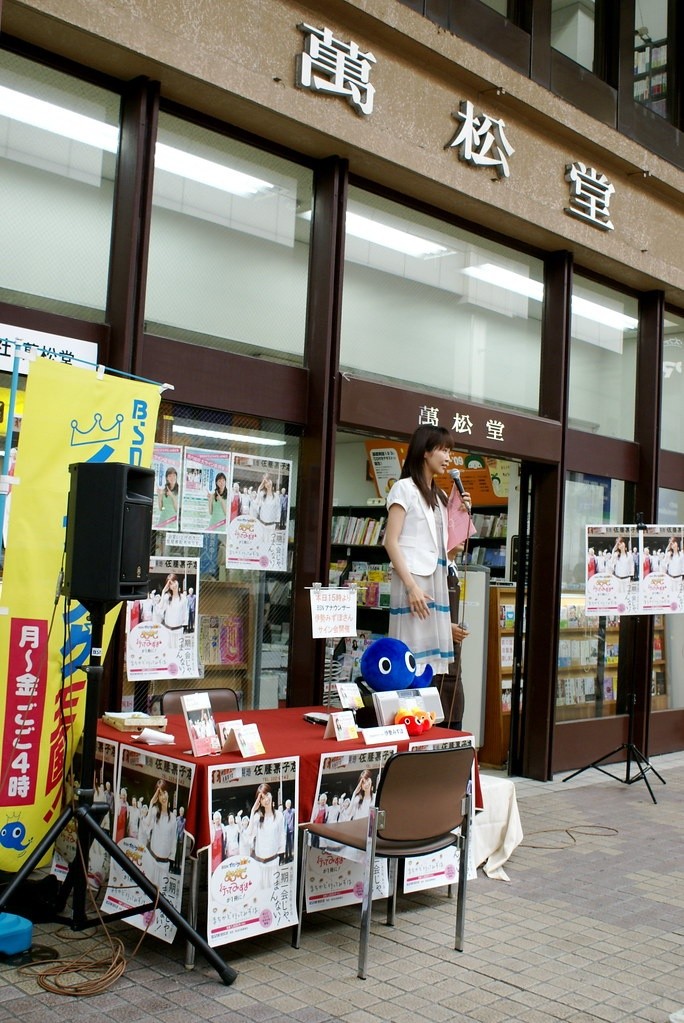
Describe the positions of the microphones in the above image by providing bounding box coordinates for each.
[450,467,471,511]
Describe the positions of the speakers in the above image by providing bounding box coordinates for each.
[65,463,156,611]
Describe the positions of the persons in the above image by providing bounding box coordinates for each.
[588,537,639,582]
[643,537,684,582]
[64,772,79,806]
[312,770,375,852]
[211,784,295,876]
[428,539,465,731]
[230,473,288,532]
[149,695,161,716]
[384,425,471,675]
[262,580,271,644]
[188,709,217,740]
[93,770,114,830]
[116,779,186,892]
[157,466,179,529]
[130,574,196,636]
[207,473,227,532]
[187,468,201,482]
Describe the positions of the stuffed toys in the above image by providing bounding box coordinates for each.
[361,638,433,692]
[394,706,436,736]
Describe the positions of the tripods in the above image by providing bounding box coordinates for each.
[0,615,238,988]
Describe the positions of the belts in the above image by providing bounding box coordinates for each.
[614,573,631,579]
[259,519,274,526]
[165,624,182,630]
[668,573,682,578]
[255,853,277,863]
[149,848,168,862]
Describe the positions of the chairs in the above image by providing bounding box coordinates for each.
[159,686,241,715]
[296,745,478,979]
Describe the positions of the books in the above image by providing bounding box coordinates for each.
[102,711,168,733]
[260,520,295,700]
[500,604,666,712]
[324,513,507,708]
[180,692,221,757]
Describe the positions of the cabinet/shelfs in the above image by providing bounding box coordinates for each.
[261,505,508,642]
[148,580,254,716]
[480,585,667,768]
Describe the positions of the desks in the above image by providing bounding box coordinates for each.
[97,706,474,968]
